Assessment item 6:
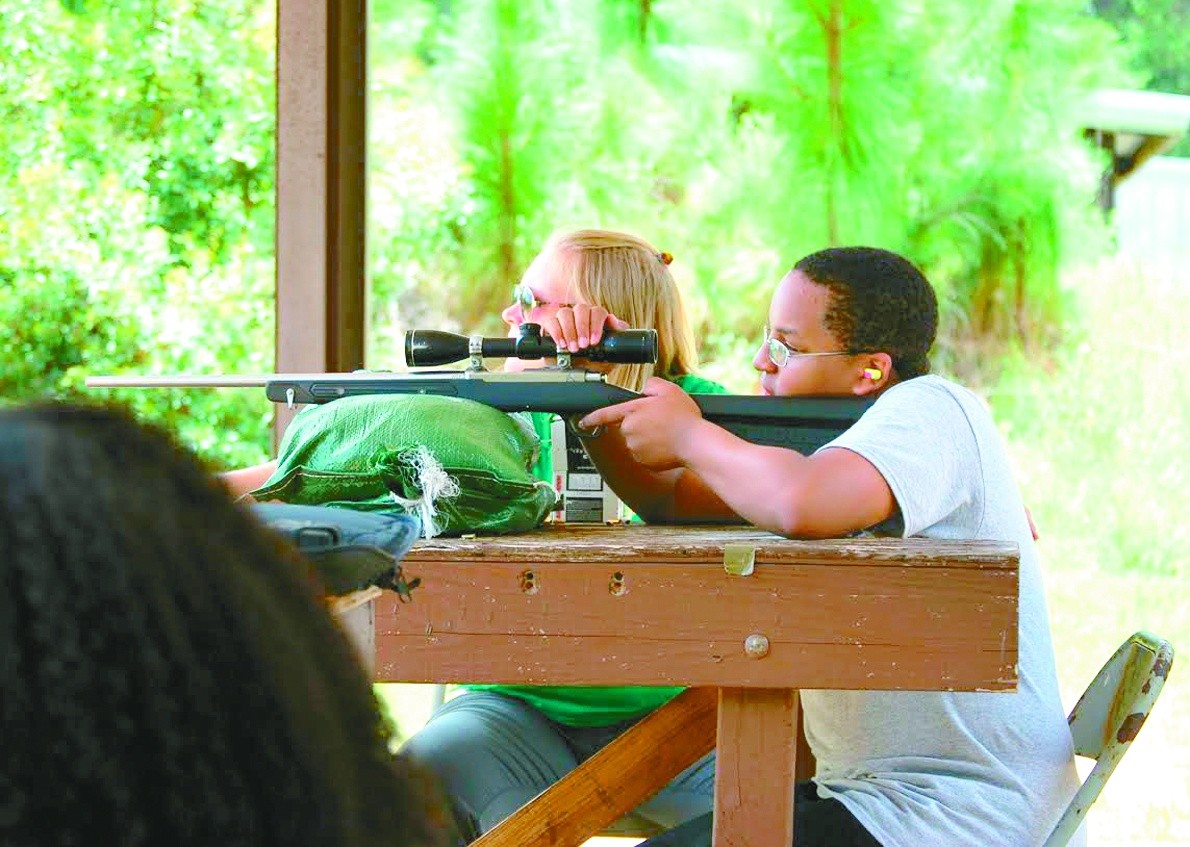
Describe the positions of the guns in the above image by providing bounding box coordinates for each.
[83,326,878,457]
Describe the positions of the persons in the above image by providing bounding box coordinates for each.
[215,229,735,847]
[0,388,484,847]
[537,247,1085,847]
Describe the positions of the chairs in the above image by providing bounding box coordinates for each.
[1035,625,1180,847]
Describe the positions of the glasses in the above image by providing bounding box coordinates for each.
[762,326,875,366]
[509,284,584,324]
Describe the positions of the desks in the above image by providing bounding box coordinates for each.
[357,517,1029,847]
